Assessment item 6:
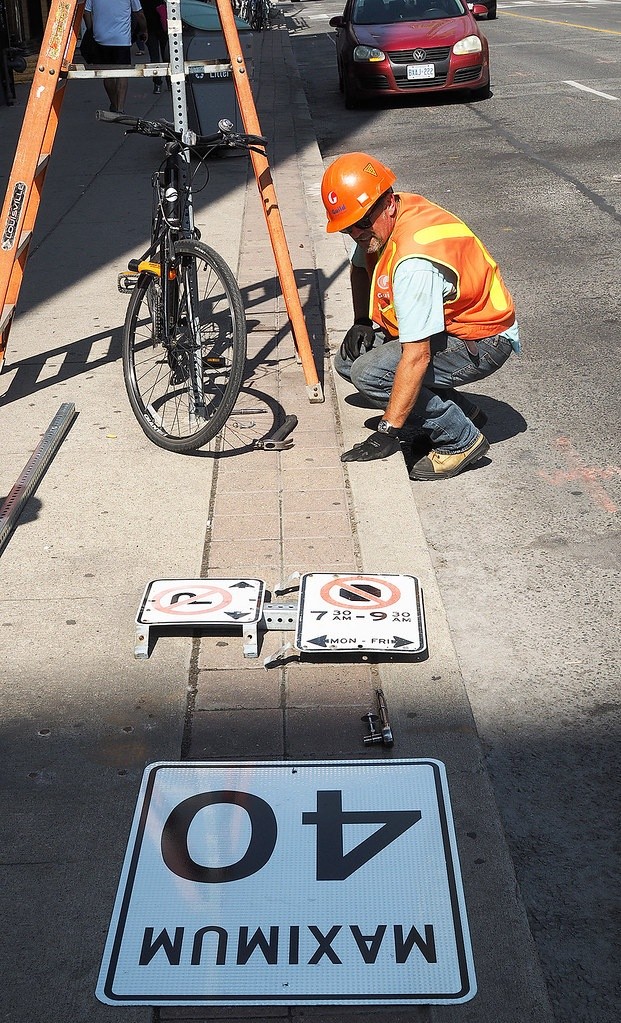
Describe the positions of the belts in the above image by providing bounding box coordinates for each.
[464,337,481,357]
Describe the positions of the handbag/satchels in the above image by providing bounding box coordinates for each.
[156,4,167,32]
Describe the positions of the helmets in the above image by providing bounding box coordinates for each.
[320,151,396,233]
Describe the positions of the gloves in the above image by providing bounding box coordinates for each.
[340,424,400,463]
[338,320,375,361]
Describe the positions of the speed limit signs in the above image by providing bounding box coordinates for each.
[94,756,478,1007]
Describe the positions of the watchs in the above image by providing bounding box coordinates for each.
[377,419,400,437]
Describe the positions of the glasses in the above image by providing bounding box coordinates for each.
[339,191,391,234]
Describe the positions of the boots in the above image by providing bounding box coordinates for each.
[407,432,490,481]
[468,406,487,431]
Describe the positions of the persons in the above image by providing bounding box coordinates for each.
[83,0,147,114]
[139,0,172,94]
[321,152,521,480]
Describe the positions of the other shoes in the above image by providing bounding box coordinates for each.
[153,83,162,94]
[135,50,145,55]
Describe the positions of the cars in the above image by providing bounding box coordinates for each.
[465,0,497,20]
[329,0,490,112]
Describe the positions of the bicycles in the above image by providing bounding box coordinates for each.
[231,0,274,33]
[92,109,271,454]
